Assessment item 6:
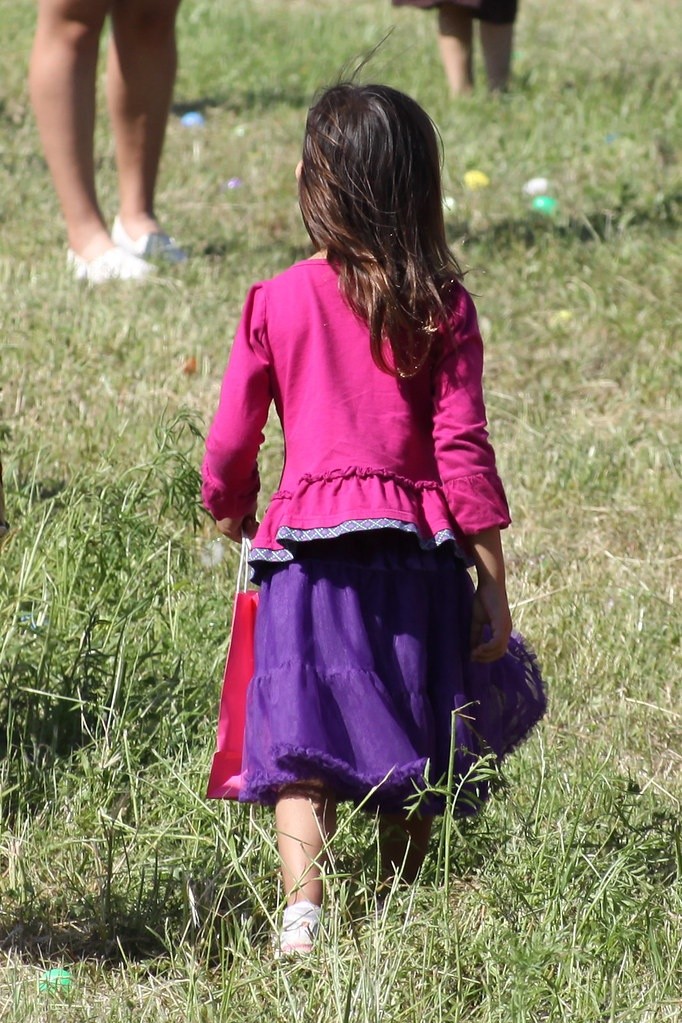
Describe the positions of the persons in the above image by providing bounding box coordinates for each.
[29,0,189,289]
[198,80,548,971]
[392,1,522,102]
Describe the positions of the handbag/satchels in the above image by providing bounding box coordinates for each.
[206,528,259,803]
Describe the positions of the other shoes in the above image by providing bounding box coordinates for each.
[274,901,325,962]
[108,215,192,266]
[66,247,158,283]
